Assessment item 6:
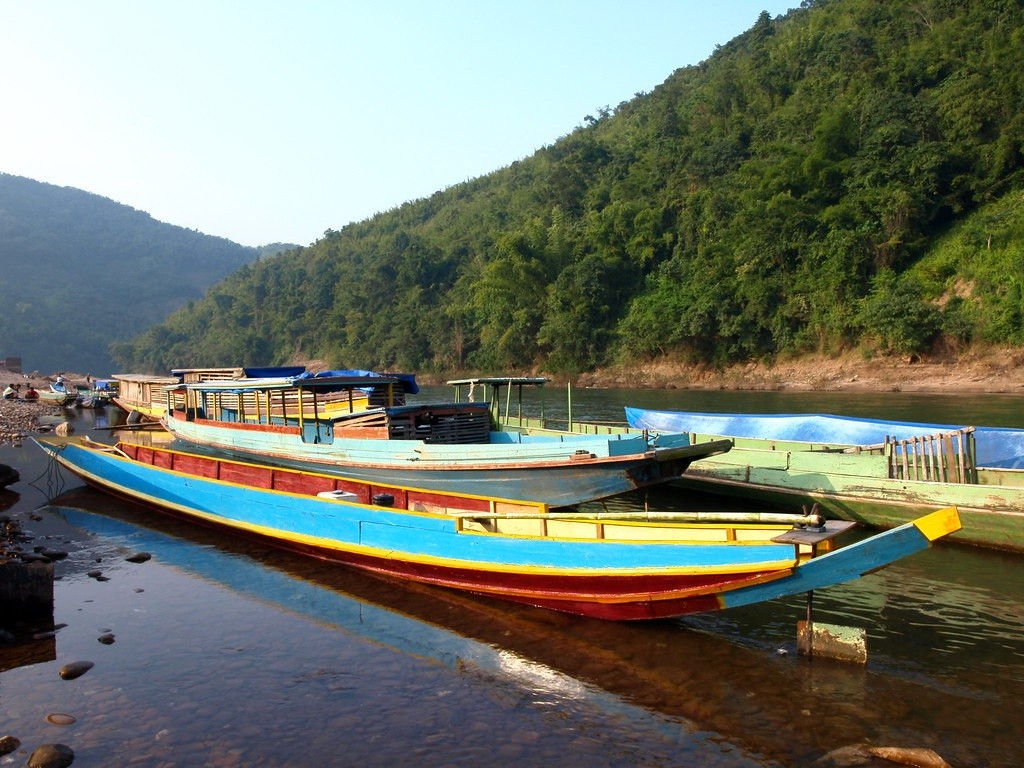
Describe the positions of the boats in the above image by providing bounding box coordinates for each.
[32,484,954,768]
[115,376,736,508]
[34,389,77,405]
[31,434,962,620]
[624,405,1024,471]
[447,379,1024,551]
[50,384,107,409]
[111,367,419,422]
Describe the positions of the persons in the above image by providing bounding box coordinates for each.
[55,375,64,386]
[3,383,21,401]
[25,387,39,402]
[87,373,90,384]
